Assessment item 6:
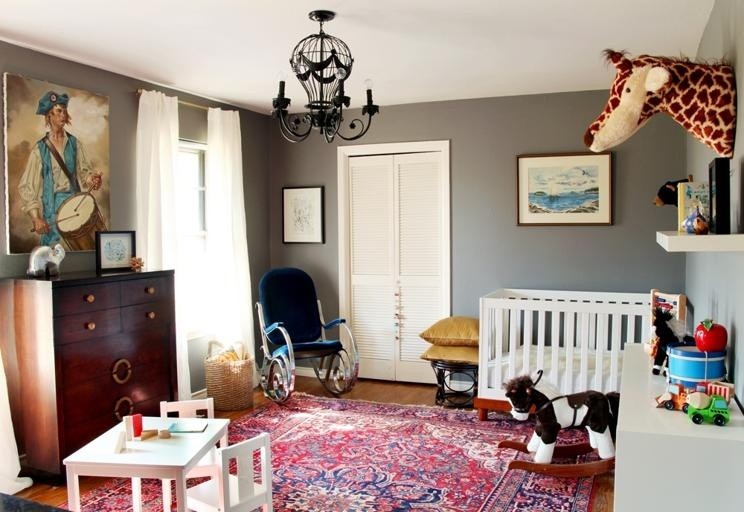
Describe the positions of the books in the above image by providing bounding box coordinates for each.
[677,183,712,232]
[158,416,208,432]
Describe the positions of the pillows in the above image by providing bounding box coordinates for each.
[419,344,478,365]
[418,316,479,346]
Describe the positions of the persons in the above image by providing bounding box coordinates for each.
[20,91,103,248]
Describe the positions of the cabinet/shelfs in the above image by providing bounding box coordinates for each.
[0,267,180,487]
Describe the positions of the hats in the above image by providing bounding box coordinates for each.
[34,90,70,116]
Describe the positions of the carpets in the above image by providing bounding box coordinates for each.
[54,391,602,512]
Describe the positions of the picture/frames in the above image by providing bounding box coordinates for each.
[515,150,614,227]
[94,230,136,274]
[280,185,325,244]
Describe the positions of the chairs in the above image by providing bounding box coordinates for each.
[253,266,358,404]
[181,432,273,512]
[157,397,218,512]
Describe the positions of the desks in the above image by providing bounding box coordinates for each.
[61,415,230,511]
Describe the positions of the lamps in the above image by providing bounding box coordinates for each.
[271,9,379,144]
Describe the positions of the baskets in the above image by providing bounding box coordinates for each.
[204,351,253,411]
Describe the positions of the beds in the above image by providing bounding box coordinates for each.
[473,286,688,421]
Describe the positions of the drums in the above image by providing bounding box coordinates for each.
[53,191,108,251]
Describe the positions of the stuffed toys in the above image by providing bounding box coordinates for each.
[652,180,689,206]
[653,309,699,376]
[584,49,737,156]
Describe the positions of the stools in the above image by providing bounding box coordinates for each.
[430,360,478,409]
